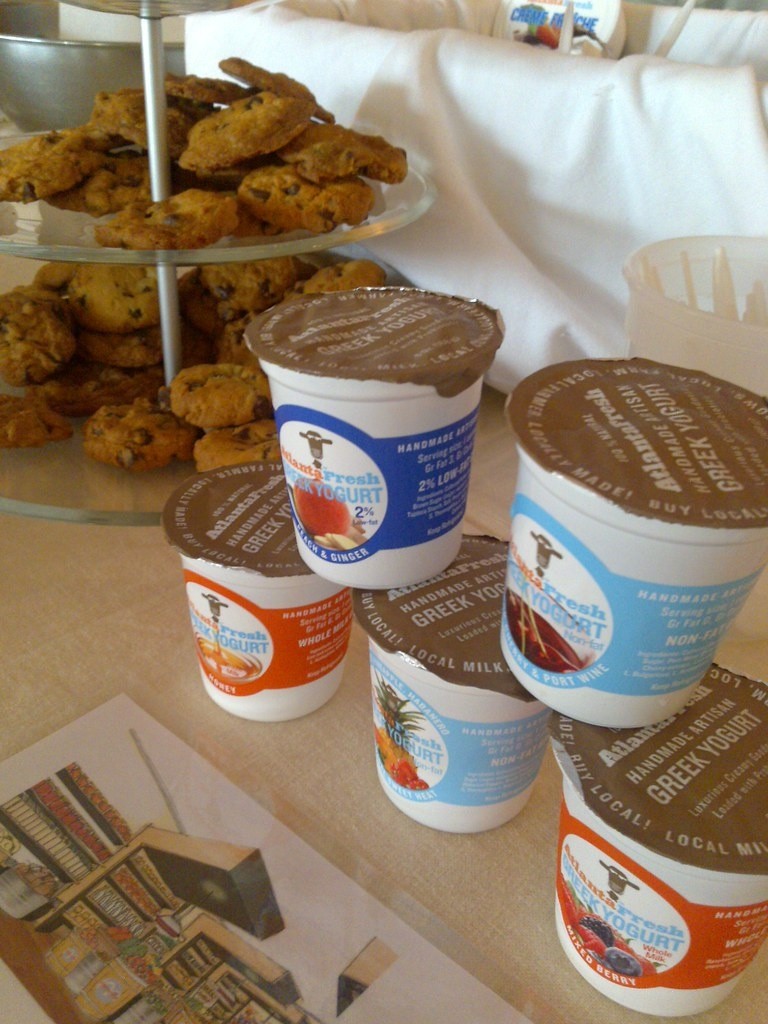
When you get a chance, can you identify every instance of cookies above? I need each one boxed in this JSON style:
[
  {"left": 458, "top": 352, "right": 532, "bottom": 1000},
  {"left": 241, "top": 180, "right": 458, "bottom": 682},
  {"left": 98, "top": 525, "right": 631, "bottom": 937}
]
[
  {"left": 0, "top": 258, "right": 391, "bottom": 476},
  {"left": 0, "top": 54, "right": 407, "bottom": 250}
]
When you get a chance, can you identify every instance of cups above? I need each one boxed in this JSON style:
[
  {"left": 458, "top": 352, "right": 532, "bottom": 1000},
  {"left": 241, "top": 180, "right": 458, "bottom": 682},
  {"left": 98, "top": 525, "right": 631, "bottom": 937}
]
[{"left": 623, "top": 237, "right": 768, "bottom": 644}]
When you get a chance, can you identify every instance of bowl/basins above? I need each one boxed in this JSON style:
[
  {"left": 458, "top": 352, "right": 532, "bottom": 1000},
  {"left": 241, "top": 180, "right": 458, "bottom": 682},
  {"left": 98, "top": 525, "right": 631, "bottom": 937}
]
[{"left": 0, "top": 34, "right": 186, "bottom": 133}]
